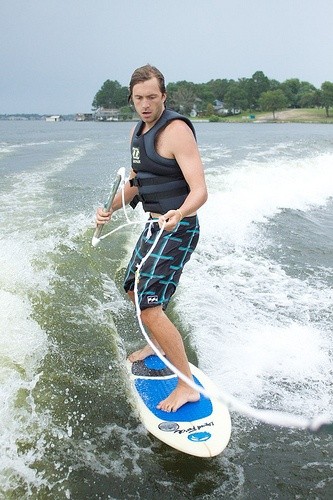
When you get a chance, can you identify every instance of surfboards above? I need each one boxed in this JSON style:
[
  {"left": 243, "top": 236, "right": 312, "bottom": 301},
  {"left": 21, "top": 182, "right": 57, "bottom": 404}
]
[{"left": 106, "top": 286, "right": 233, "bottom": 459}]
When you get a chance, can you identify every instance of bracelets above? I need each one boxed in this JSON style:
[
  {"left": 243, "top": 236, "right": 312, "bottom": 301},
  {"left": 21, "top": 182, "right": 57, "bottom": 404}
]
[{"left": 175, "top": 207, "right": 186, "bottom": 222}]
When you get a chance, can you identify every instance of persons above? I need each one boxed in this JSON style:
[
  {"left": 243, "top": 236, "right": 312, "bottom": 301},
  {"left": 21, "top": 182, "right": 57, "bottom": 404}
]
[{"left": 96, "top": 64, "right": 209, "bottom": 413}]
